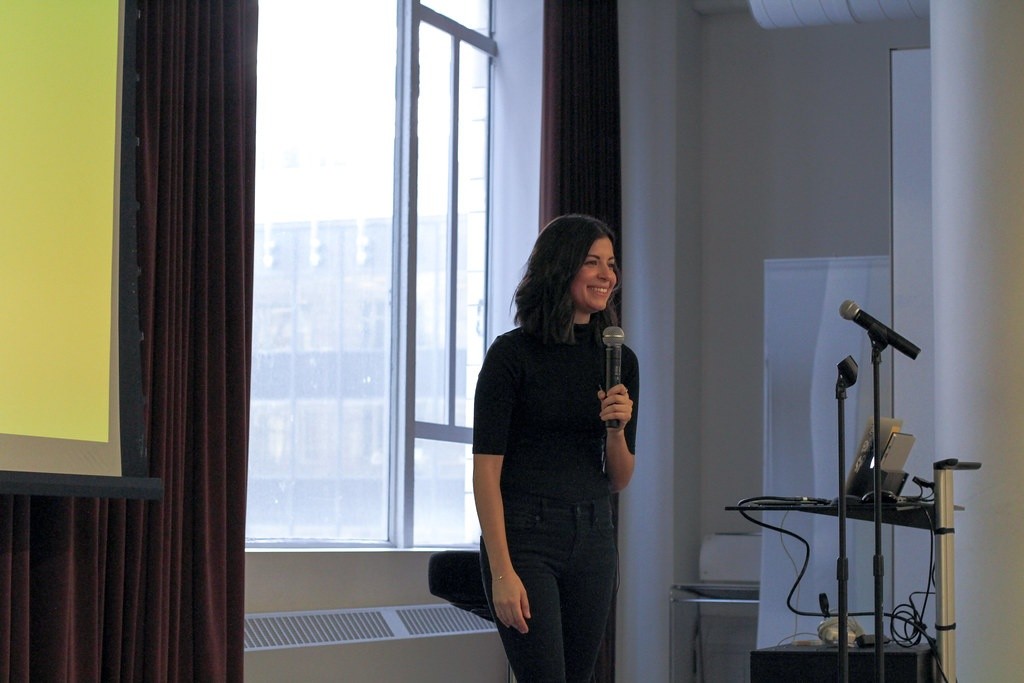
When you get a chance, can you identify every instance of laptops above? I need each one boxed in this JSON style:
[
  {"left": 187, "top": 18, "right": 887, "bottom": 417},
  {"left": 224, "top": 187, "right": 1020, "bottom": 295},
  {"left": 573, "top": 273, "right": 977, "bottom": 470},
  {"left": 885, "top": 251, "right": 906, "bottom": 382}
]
[{"left": 752, "top": 417, "right": 904, "bottom": 506}]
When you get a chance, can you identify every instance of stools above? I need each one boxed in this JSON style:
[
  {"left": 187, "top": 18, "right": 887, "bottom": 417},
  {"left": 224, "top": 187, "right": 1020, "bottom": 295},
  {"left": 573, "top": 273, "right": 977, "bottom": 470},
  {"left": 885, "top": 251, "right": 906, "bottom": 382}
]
[{"left": 428, "top": 550, "right": 517, "bottom": 683}]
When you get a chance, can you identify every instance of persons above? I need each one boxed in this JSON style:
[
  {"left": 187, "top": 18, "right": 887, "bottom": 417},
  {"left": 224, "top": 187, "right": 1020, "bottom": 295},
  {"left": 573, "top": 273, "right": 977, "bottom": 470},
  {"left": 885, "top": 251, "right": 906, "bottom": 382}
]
[{"left": 472, "top": 213, "right": 640, "bottom": 683}]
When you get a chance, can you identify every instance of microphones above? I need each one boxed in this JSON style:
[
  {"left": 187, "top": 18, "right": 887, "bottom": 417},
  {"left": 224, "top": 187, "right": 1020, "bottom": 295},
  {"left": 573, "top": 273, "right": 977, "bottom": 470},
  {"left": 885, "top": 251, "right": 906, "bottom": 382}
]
[
  {"left": 839, "top": 300, "right": 922, "bottom": 360},
  {"left": 602, "top": 326, "right": 625, "bottom": 428}
]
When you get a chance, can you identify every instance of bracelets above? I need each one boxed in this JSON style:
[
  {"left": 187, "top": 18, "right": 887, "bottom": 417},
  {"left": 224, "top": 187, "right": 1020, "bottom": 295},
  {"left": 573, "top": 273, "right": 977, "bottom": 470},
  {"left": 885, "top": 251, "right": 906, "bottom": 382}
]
[{"left": 492, "top": 569, "right": 514, "bottom": 580}]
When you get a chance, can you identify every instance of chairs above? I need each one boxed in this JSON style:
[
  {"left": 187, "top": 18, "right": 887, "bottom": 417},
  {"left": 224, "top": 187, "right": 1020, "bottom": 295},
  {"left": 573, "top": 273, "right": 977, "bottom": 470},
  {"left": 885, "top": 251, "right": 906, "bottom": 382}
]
[{"left": 667, "top": 532, "right": 763, "bottom": 683}]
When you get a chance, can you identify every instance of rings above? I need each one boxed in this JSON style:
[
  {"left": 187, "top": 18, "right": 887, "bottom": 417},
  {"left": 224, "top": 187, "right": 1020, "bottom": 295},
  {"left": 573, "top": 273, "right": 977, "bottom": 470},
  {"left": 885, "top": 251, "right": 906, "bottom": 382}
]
[{"left": 623, "top": 389, "right": 628, "bottom": 395}]
